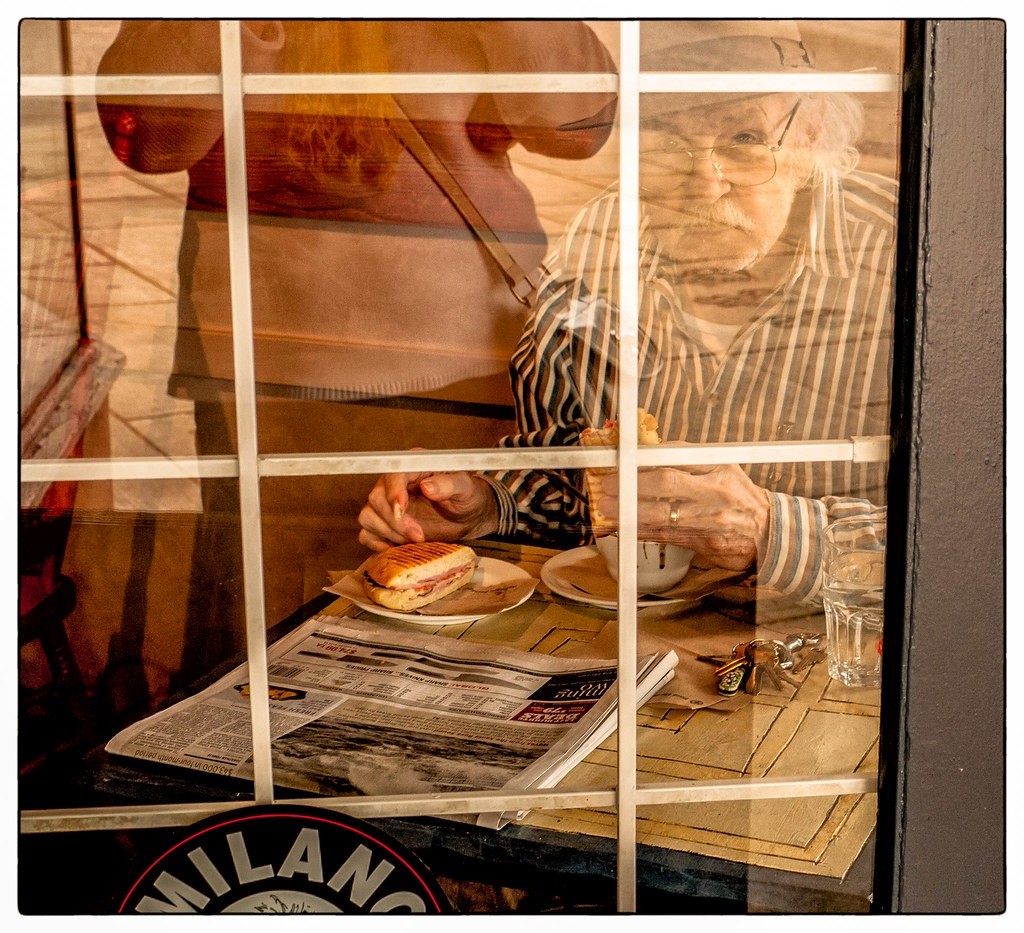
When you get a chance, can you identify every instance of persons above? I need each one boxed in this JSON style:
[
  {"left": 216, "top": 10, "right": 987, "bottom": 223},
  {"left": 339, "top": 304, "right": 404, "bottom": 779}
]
[
  {"left": 352, "top": 20, "right": 903, "bottom": 628},
  {"left": 98, "top": 21, "right": 618, "bottom": 694}
]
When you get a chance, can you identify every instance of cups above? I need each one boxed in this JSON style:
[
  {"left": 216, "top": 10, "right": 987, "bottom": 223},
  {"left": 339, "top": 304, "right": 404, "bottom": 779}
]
[
  {"left": 599, "top": 532, "right": 697, "bottom": 592},
  {"left": 818, "top": 517, "right": 886, "bottom": 688}
]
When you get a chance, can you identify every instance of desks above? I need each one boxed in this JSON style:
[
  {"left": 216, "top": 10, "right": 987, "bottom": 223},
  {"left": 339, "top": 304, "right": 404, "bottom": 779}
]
[{"left": 87, "top": 540, "right": 882, "bottom": 914}]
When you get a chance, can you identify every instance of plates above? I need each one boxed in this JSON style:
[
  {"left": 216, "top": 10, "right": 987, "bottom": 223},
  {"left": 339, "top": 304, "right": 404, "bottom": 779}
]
[
  {"left": 356, "top": 557, "right": 537, "bottom": 625},
  {"left": 541, "top": 545, "right": 684, "bottom": 611}
]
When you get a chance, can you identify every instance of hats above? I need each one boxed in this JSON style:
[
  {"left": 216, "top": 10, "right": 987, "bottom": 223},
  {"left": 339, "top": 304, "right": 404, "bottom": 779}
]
[{"left": 636, "top": 21, "right": 817, "bottom": 122}]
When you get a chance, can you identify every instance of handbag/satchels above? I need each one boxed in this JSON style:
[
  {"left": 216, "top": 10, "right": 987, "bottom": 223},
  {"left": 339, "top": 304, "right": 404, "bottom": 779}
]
[{"left": 524, "top": 279, "right": 660, "bottom": 430}]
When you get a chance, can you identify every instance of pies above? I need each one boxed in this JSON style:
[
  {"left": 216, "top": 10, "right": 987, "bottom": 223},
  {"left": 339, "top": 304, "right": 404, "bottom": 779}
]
[
  {"left": 579, "top": 408, "right": 663, "bottom": 537},
  {"left": 361, "top": 541, "right": 477, "bottom": 610},
  {"left": 273, "top": 20, "right": 398, "bottom": 199}
]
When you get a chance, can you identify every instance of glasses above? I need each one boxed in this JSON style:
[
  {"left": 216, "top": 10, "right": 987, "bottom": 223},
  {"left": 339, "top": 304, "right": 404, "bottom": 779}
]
[{"left": 636, "top": 97, "right": 806, "bottom": 195}]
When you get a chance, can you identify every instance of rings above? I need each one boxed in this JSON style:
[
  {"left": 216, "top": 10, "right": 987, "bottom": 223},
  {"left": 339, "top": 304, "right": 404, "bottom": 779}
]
[{"left": 669, "top": 502, "right": 682, "bottom": 526}]
